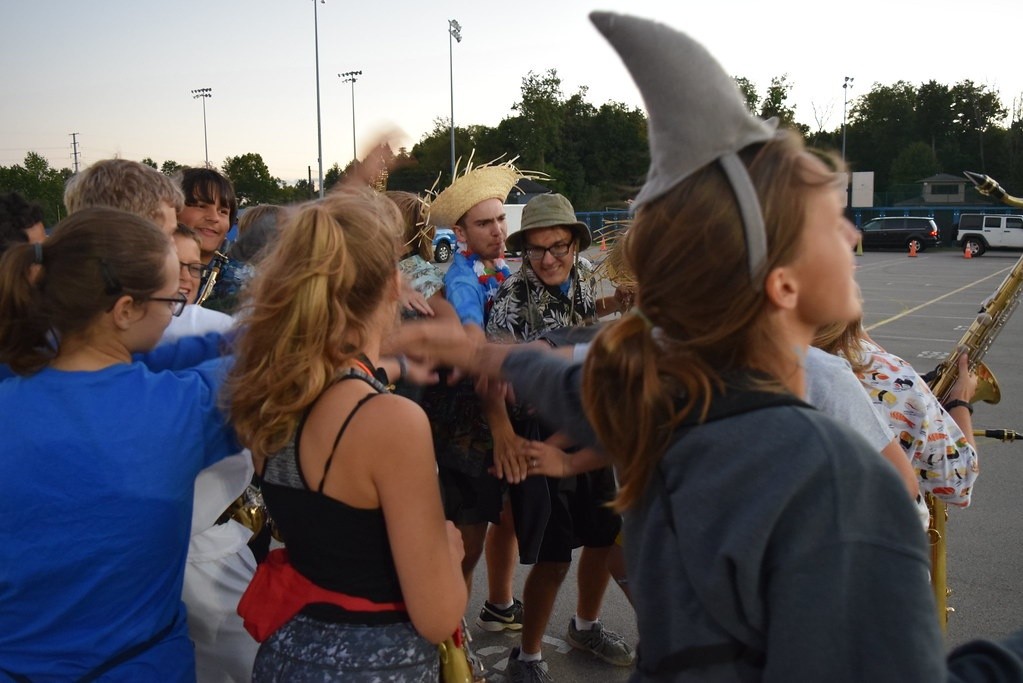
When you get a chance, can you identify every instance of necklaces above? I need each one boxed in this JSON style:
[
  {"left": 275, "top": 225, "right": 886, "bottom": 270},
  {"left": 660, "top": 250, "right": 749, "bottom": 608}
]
[{"left": 348, "top": 359, "right": 375, "bottom": 377}]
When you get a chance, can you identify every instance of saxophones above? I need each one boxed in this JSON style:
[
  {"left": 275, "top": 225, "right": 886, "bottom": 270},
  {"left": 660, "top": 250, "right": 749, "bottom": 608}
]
[
  {"left": 438, "top": 615, "right": 487, "bottom": 683},
  {"left": 929, "top": 170, "right": 1023, "bottom": 646},
  {"left": 225, "top": 484, "right": 287, "bottom": 543},
  {"left": 196, "top": 238, "right": 231, "bottom": 307}
]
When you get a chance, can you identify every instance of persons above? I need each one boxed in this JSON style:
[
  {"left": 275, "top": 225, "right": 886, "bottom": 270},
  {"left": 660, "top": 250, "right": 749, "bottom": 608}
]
[
  {"left": 0, "top": 156, "right": 636, "bottom": 683},
  {"left": 0, "top": 208, "right": 258, "bottom": 683},
  {"left": 426, "top": 8, "right": 980, "bottom": 683}
]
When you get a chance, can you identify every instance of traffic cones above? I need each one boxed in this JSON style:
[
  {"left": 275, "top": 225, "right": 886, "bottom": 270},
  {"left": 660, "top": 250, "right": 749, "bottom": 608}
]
[
  {"left": 908, "top": 240, "right": 917, "bottom": 257},
  {"left": 600, "top": 236, "right": 607, "bottom": 250},
  {"left": 963, "top": 240, "right": 972, "bottom": 259}
]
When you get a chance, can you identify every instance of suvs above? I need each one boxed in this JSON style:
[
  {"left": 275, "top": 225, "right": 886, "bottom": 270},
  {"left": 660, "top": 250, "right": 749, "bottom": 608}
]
[
  {"left": 854, "top": 217, "right": 943, "bottom": 252},
  {"left": 957, "top": 213, "right": 1023, "bottom": 257}
]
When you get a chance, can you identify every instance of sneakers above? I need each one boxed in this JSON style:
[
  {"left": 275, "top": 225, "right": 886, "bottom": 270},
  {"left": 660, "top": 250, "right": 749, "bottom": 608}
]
[
  {"left": 566, "top": 616, "right": 636, "bottom": 665},
  {"left": 504, "top": 646, "right": 555, "bottom": 683},
  {"left": 475, "top": 597, "right": 525, "bottom": 632}
]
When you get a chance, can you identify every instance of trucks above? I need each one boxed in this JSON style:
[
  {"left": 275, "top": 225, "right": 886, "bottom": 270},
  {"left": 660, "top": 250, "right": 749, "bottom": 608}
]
[{"left": 431, "top": 204, "right": 527, "bottom": 263}]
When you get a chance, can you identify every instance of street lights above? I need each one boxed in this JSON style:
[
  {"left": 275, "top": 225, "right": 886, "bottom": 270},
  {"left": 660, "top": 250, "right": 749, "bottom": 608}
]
[
  {"left": 312, "top": 0, "right": 326, "bottom": 199},
  {"left": 338, "top": 71, "right": 362, "bottom": 167},
  {"left": 191, "top": 88, "right": 212, "bottom": 168},
  {"left": 449, "top": 19, "right": 462, "bottom": 175}
]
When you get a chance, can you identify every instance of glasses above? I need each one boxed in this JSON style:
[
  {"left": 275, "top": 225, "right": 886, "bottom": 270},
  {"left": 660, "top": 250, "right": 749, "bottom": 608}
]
[
  {"left": 179, "top": 261, "right": 209, "bottom": 278},
  {"left": 106, "top": 291, "right": 188, "bottom": 317},
  {"left": 523, "top": 234, "right": 577, "bottom": 259}
]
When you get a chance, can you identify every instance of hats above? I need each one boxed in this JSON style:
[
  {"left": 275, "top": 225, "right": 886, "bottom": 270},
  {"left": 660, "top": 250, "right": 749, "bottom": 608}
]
[
  {"left": 404, "top": 149, "right": 557, "bottom": 246},
  {"left": 505, "top": 194, "right": 592, "bottom": 253}
]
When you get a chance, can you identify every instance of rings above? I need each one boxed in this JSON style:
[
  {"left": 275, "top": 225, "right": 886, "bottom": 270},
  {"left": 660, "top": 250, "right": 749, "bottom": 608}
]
[{"left": 533, "top": 459, "right": 536, "bottom": 466}]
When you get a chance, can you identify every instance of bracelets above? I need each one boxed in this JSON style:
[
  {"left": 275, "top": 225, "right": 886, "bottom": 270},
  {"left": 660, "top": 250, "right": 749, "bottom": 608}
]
[
  {"left": 944, "top": 399, "right": 974, "bottom": 416},
  {"left": 397, "top": 353, "right": 408, "bottom": 384}
]
